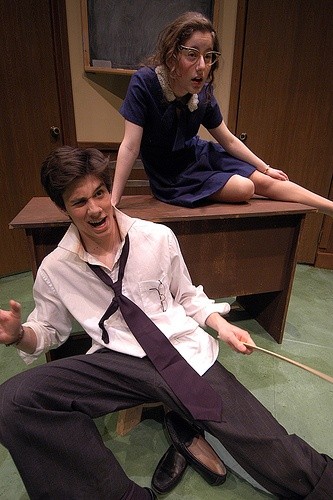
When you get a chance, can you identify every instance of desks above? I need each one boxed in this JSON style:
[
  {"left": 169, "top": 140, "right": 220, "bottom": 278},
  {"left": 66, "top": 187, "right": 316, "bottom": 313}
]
[{"left": 11, "top": 194, "right": 317, "bottom": 363}]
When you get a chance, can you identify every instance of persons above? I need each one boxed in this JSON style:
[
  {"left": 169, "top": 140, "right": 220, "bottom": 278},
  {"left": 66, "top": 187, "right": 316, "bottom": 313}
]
[
  {"left": 1, "top": 143, "right": 333, "bottom": 500},
  {"left": 110, "top": 13, "right": 333, "bottom": 217}
]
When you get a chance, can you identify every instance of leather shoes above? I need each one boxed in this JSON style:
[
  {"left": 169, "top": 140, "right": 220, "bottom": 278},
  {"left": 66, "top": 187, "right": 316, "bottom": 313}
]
[
  {"left": 162, "top": 409, "right": 227, "bottom": 486},
  {"left": 151, "top": 446, "right": 188, "bottom": 495}
]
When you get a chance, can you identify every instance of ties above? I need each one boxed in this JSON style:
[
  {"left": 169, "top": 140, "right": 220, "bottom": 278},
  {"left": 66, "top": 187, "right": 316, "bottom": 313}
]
[{"left": 86, "top": 233, "right": 221, "bottom": 422}]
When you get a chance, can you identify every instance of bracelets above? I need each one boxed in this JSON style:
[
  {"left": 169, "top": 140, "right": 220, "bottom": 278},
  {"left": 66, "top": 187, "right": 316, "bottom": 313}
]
[
  {"left": 4, "top": 324, "right": 25, "bottom": 347},
  {"left": 262, "top": 165, "right": 270, "bottom": 174}
]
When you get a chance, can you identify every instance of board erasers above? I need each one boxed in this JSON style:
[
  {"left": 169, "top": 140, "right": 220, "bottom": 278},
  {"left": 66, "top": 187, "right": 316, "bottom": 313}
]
[{"left": 92, "top": 60, "right": 111, "bottom": 68}]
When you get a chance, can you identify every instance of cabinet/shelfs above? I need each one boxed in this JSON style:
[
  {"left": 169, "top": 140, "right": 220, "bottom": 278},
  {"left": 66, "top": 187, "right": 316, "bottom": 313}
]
[
  {"left": 227, "top": 0, "right": 333, "bottom": 269},
  {"left": 0, "top": 0, "right": 75, "bottom": 278}
]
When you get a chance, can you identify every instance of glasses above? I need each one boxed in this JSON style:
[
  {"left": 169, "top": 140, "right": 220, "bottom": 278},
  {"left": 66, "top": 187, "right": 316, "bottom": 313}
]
[{"left": 177, "top": 44, "right": 222, "bottom": 66}]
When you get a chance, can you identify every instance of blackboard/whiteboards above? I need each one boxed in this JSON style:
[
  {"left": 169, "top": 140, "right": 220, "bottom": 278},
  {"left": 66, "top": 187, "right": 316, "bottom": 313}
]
[{"left": 80, "top": 0, "right": 220, "bottom": 76}]
